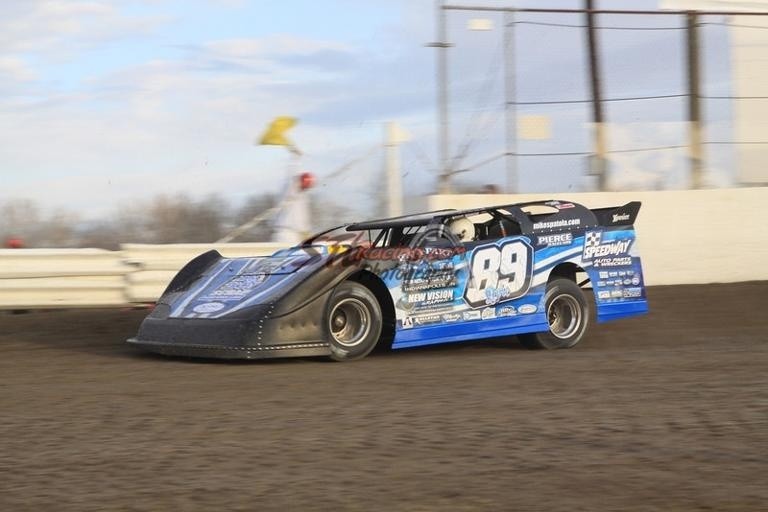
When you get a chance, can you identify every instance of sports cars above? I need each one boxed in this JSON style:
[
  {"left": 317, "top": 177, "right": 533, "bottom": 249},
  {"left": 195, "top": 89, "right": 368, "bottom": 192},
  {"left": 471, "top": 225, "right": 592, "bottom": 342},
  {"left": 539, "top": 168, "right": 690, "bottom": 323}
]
[{"left": 122, "top": 196, "right": 649, "bottom": 365}]
